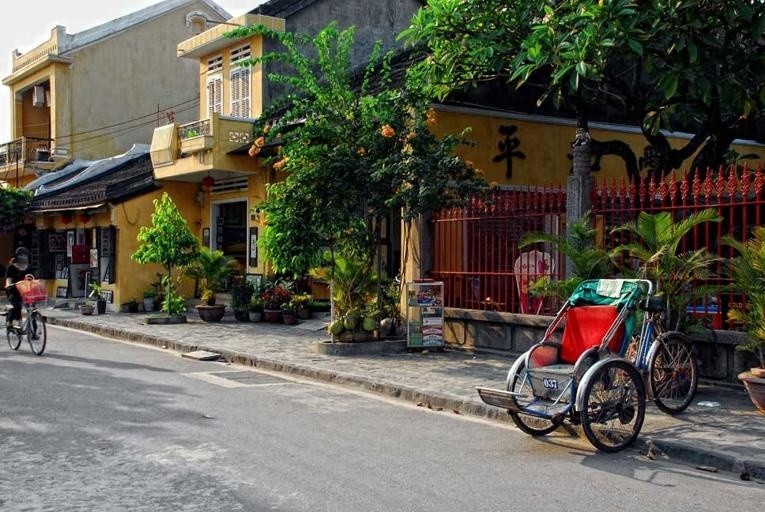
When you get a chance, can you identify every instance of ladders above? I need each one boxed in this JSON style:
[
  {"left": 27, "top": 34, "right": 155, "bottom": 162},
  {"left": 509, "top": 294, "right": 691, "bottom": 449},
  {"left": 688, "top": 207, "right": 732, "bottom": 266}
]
[{"left": 31, "top": 229, "right": 40, "bottom": 279}]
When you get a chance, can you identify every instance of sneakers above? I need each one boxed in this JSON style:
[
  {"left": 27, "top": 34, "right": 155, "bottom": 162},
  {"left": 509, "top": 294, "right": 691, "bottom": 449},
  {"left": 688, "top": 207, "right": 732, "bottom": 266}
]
[{"left": 12, "top": 319, "right": 21, "bottom": 328}]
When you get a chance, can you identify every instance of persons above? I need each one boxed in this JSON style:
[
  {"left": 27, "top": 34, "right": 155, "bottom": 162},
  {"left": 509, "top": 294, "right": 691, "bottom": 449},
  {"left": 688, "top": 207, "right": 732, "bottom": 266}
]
[
  {"left": 49, "top": 228, "right": 109, "bottom": 291},
  {"left": 3, "top": 246, "right": 41, "bottom": 341}
]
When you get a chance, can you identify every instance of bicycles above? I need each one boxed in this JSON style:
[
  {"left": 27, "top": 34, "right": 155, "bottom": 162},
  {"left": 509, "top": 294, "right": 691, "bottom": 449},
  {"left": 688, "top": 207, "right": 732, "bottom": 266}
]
[{"left": 4, "top": 283, "right": 46, "bottom": 356}]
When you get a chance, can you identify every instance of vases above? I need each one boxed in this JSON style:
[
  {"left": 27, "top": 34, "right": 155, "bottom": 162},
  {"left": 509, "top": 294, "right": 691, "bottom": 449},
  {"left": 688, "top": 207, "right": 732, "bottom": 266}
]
[
  {"left": 235, "top": 309, "right": 311, "bottom": 325},
  {"left": 128, "top": 303, "right": 139, "bottom": 313},
  {"left": 97, "top": 300, "right": 106, "bottom": 314},
  {"left": 232, "top": 281, "right": 313, "bottom": 314},
  {"left": 79, "top": 306, "right": 96, "bottom": 315}
]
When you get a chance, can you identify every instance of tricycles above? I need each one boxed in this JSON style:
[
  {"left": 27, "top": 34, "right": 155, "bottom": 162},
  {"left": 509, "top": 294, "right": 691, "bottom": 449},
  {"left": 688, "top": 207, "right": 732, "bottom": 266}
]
[{"left": 475, "top": 277, "right": 700, "bottom": 454}]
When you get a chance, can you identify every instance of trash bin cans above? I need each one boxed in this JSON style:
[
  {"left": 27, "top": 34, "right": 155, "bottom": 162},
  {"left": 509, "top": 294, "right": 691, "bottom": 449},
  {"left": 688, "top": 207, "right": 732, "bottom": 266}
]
[{"left": 686, "top": 292, "right": 722, "bottom": 329}]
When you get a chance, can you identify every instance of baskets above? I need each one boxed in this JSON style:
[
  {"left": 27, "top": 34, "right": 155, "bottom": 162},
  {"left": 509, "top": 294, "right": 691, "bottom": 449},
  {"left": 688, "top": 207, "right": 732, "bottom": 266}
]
[{"left": 14, "top": 274, "right": 48, "bottom": 304}]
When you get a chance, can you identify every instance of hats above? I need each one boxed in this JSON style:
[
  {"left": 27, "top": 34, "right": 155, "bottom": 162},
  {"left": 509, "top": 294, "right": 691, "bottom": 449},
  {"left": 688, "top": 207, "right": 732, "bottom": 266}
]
[{"left": 15, "top": 247, "right": 30, "bottom": 255}]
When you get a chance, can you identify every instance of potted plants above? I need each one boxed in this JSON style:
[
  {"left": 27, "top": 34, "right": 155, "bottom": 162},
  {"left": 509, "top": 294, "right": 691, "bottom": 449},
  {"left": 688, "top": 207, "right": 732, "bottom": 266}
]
[
  {"left": 719, "top": 226, "right": 765, "bottom": 413},
  {"left": 179, "top": 247, "right": 242, "bottom": 323},
  {"left": 144, "top": 291, "right": 157, "bottom": 312},
  {"left": 610, "top": 209, "right": 723, "bottom": 399}
]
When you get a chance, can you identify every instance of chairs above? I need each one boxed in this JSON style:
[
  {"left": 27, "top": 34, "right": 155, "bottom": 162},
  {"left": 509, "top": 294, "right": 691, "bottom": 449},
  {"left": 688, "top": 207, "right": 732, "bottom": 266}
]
[{"left": 525, "top": 305, "right": 624, "bottom": 404}]
